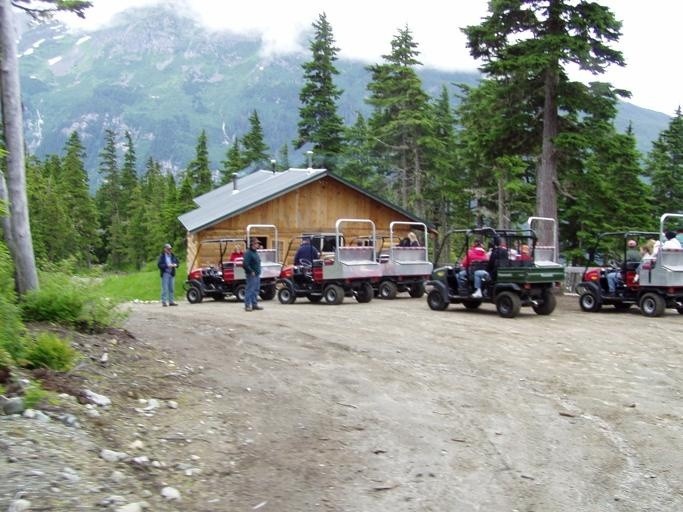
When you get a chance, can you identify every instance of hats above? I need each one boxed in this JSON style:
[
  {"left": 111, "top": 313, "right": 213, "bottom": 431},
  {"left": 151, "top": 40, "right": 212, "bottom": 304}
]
[
  {"left": 250, "top": 237, "right": 262, "bottom": 245},
  {"left": 627, "top": 239, "right": 637, "bottom": 247},
  {"left": 165, "top": 244, "right": 172, "bottom": 249}
]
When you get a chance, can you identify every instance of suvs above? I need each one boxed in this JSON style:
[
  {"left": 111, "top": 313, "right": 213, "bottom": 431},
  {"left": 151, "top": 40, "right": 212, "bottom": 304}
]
[
  {"left": 422, "top": 218, "right": 564, "bottom": 318},
  {"left": 348, "top": 221, "right": 432, "bottom": 300},
  {"left": 183, "top": 224, "right": 282, "bottom": 304},
  {"left": 276, "top": 219, "right": 383, "bottom": 304},
  {"left": 575, "top": 213, "right": 683, "bottom": 316}
]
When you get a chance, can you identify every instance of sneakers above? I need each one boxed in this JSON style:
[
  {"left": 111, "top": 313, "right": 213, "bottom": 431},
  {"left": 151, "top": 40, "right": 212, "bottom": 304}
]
[
  {"left": 471, "top": 290, "right": 484, "bottom": 299},
  {"left": 163, "top": 302, "right": 179, "bottom": 306},
  {"left": 245, "top": 304, "right": 264, "bottom": 311}
]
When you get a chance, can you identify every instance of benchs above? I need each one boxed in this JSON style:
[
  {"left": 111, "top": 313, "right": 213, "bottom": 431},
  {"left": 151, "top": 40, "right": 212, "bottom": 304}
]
[
  {"left": 325, "top": 240, "right": 372, "bottom": 265},
  {"left": 391, "top": 245, "right": 427, "bottom": 263},
  {"left": 617, "top": 261, "right": 639, "bottom": 287},
  {"left": 322, "top": 252, "right": 336, "bottom": 265},
  {"left": 489, "top": 258, "right": 534, "bottom": 284},
  {"left": 467, "top": 260, "right": 488, "bottom": 283},
  {"left": 233, "top": 249, "right": 282, "bottom": 280},
  {"left": 638, "top": 247, "right": 683, "bottom": 287},
  {"left": 378, "top": 253, "right": 389, "bottom": 264}
]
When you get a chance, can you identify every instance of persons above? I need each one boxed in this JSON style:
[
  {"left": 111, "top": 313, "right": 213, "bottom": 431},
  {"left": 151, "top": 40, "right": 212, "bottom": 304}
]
[
  {"left": 293, "top": 238, "right": 320, "bottom": 266},
  {"left": 605, "top": 228, "right": 682, "bottom": 297},
  {"left": 240, "top": 237, "right": 264, "bottom": 312},
  {"left": 156, "top": 243, "right": 180, "bottom": 308},
  {"left": 459, "top": 237, "right": 534, "bottom": 298},
  {"left": 408, "top": 232, "right": 419, "bottom": 246},
  {"left": 228, "top": 245, "right": 244, "bottom": 261}
]
